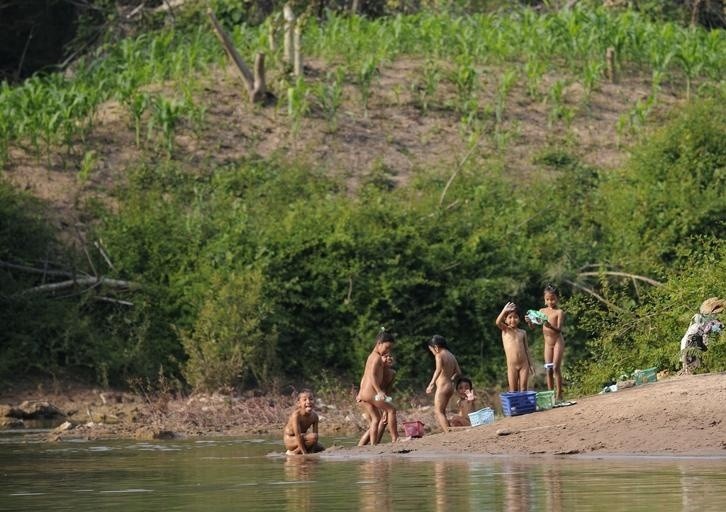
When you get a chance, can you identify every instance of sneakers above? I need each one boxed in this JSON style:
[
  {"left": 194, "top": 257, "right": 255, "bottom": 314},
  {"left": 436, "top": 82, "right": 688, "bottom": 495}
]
[{"left": 552, "top": 399, "right": 576, "bottom": 408}]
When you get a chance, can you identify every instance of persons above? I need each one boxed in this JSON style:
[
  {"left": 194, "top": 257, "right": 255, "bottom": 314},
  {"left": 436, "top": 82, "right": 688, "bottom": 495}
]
[
  {"left": 495, "top": 301, "right": 536, "bottom": 393},
  {"left": 283, "top": 388, "right": 326, "bottom": 456},
  {"left": 356, "top": 330, "right": 399, "bottom": 446},
  {"left": 423, "top": 335, "right": 477, "bottom": 435},
  {"left": 525, "top": 285, "right": 565, "bottom": 403}
]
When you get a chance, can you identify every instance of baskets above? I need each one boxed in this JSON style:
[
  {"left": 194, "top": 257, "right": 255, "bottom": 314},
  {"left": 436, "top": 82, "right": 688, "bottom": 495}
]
[
  {"left": 536, "top": 391, "right": 555, "bottom": 412},
  {"left": 631, "top": 367, "right": 657, "bottom": 385},
  {"left": 468, "top": 407, "right": 495, "bottom": 427},
  {"left": 499, "top": 390, "right": 537, "bottom": 416},
  {"left": 402, "top": 421, "right": 425, "bottom": 437}
]
